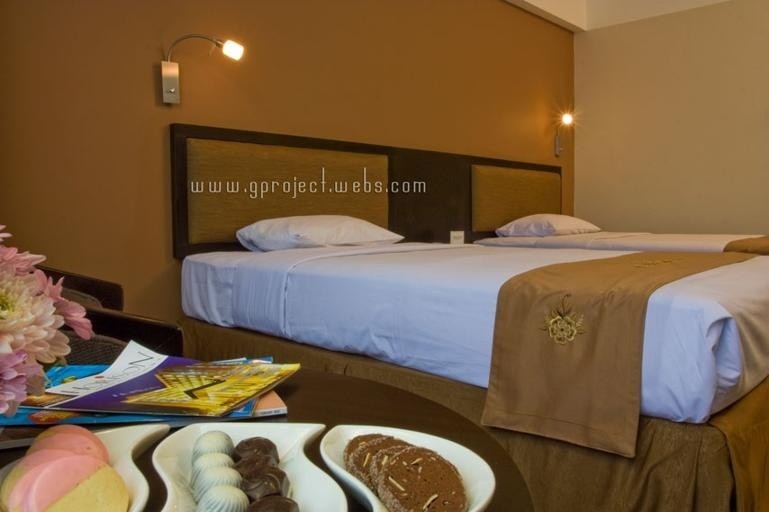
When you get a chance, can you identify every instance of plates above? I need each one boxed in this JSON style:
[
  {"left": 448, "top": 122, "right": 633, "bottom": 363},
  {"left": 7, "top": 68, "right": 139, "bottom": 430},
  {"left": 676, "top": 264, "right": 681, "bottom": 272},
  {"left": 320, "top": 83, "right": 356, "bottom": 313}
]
[
  {"left": 150, "top": 421, "right": 348, "bottom": 512},
  {"left": 1, "top": 424, "right": 170, "bottom": 512},
  {"left": 319, "top": 421, "right": 497, "bottom": 512}
]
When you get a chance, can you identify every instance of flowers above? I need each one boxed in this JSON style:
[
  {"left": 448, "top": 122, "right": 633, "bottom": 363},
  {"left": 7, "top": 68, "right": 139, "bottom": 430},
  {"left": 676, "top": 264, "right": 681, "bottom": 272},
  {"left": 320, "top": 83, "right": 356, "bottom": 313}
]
[{"left": 0, "top": 224, "right": 96, "bottom": 418}]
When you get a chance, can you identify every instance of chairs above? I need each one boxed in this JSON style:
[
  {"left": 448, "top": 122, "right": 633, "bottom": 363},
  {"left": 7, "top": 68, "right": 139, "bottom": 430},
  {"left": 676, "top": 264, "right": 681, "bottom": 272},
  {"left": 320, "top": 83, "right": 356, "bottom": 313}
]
[{"left": 29, "top": 264, "right": 184, "bottom": 372}]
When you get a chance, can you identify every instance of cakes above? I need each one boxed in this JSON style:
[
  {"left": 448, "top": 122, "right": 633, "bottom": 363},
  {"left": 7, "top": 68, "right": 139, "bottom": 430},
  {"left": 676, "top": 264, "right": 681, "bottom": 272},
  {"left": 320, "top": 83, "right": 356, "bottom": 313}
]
[
  {"left": 189, "top": 430, "right": 301, "bottom": 512},
  {"left": 0, "top": 424, "right": 129, "bottom": 512}
]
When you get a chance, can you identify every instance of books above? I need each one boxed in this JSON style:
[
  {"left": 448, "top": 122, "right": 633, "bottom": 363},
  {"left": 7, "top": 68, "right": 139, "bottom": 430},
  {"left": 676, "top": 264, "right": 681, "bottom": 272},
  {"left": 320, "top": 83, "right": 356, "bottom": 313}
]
[{"left": 0, "top": 338, "right": 303, "bottom": 449}]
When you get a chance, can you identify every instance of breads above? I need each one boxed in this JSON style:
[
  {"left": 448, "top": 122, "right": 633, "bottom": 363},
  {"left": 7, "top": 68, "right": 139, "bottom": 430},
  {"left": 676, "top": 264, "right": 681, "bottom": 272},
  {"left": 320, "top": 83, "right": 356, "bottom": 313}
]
[{"left": 343, "top": 434, "right": 469, "bottom": 512}]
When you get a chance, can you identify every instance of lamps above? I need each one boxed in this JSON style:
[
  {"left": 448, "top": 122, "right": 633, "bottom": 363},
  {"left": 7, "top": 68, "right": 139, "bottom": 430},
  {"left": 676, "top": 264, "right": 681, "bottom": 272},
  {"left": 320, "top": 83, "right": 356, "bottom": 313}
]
[
  {"left": 161, "top": 34, "right": 244, "bottom": 103},
  {"left": 555, "top": 114, "right": 572, "bottom": 157}
]
